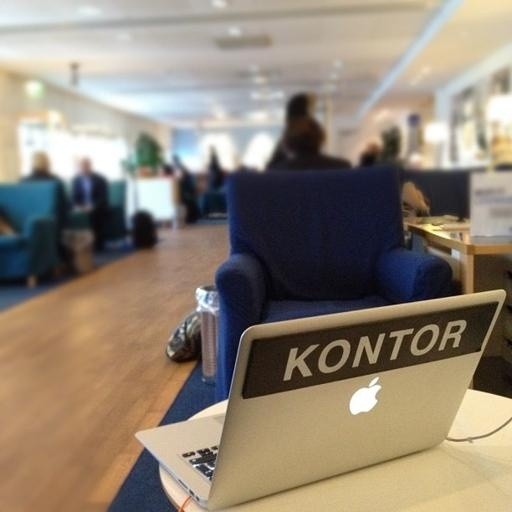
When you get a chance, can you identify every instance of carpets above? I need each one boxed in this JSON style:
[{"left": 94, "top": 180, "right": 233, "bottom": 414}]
[
  {"left": 107, "top": 355, "right": 213, "bottom": 512},
  {"left": 0, "top": 240, "right": 136, "bottom": 312}
]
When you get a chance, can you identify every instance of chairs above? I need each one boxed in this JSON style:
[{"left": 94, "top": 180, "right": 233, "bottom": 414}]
[
  {"left": 0, "top": 179, "right": 125, "bottom": 287},
  {"left": 214, "top": 162, "right": 454, "bottom": 404}
]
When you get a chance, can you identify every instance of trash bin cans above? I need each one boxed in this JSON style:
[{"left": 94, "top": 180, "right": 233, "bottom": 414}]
[{"left": 63, "top": 228, "right": 93, "bottom": 274}]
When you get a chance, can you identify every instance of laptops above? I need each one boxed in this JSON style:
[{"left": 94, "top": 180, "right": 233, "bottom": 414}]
[{"left": 134, "top": 289, "right": 507, "bottom": 510}]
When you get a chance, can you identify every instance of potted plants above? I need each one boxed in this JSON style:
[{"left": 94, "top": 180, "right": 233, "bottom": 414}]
[{"left": 135, "top": 132, "right": 164, "bottom": 177}]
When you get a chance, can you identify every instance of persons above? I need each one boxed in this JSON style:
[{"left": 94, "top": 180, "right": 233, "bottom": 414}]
[
  {"left": 0, "top": 150, "right": 112, "bottom": 288},
  {"left": 265, "top": 92, "right": 404, "bottom": 173},
  {"left": 138, "top": 144, "right": 222, "bottom": 224}
]
